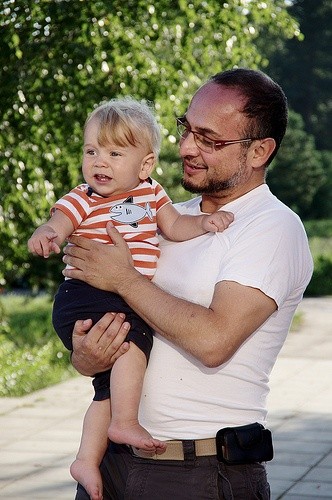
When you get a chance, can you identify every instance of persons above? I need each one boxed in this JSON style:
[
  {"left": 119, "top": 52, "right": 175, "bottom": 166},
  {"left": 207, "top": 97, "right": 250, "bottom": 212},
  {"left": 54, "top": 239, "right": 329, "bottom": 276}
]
[
  {"left": 60, "top": 67, "right": 315, "bottom": 499},
  {"left": 25, "top": 101, "right": 234, "bottom": 499}
]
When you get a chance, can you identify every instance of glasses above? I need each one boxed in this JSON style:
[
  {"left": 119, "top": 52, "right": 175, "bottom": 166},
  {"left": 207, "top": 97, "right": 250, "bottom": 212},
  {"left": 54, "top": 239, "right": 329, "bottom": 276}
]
[{"left": 176, "top": 117, "right": 260, "bottom": 153}]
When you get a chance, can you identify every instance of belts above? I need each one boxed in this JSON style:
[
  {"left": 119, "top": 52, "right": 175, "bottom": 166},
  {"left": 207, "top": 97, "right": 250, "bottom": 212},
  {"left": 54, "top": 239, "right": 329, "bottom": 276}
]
[{"left": 126, "top": 437, "right": 217, "bottom": 460}]
